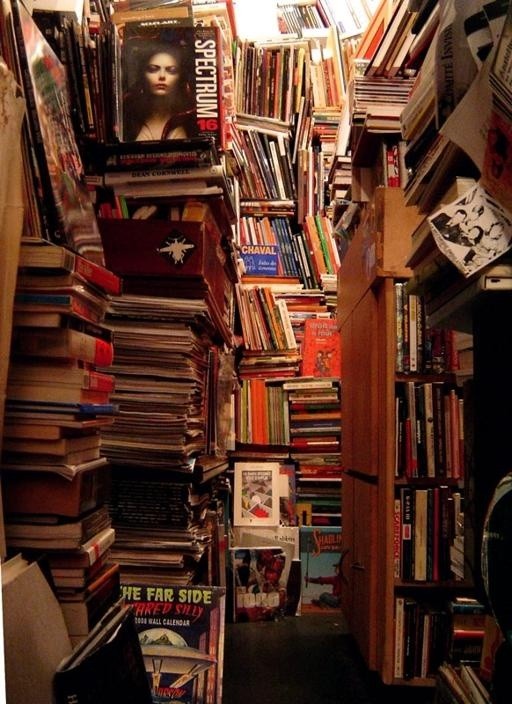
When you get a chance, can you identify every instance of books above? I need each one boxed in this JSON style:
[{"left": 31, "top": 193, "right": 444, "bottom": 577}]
[{"left": 0, "top": 0, "right": 512, "bottom": 704}]
